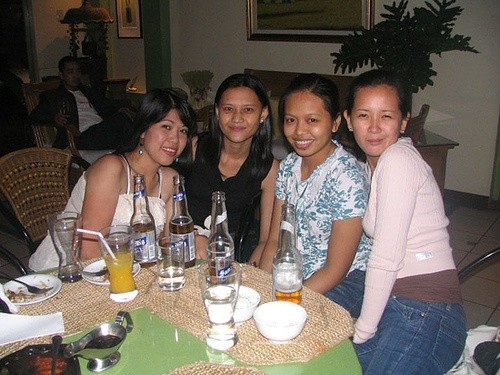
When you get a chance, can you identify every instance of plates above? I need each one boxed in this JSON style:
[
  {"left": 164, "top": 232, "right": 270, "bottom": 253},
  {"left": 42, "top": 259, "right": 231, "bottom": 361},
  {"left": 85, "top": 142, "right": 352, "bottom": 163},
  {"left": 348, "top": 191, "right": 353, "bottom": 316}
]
[
  {"left": 4, "top": 274, "right": 63, "bottom": 305},
  {"left": 81, "top": 257, "right": 141, "bottom": 286},
  {"left": 0, "top": 342, "right": 82, "bottom": 375}
]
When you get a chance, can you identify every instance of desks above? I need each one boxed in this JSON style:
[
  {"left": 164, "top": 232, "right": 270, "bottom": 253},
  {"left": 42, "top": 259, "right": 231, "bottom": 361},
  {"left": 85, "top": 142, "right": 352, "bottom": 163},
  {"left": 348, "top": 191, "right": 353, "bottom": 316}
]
[{"left": 407, "top": 129, "right": 459, "bottom": 201}]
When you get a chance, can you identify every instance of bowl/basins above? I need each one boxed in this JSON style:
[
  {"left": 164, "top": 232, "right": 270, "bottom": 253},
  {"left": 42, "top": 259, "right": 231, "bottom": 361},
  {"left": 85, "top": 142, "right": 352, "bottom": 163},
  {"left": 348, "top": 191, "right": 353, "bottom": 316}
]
[
  {"left": 253, "top": 300, "right": 308, "bottom": 341},
  {"left": 225, "top": 284, "right": 261, "bottom": 323}
]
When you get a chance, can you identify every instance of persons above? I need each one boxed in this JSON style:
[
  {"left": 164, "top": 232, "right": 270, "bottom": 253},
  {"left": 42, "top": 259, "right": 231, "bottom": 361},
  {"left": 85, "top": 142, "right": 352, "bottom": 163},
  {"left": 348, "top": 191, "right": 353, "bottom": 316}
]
[
  {"left": 27, "top": 56, "right": 139, "bottom": 150},
  {"left": 259, "top": 73, "right": 372, "bottom": 322},
  {"left": 27, "top": 86, "right": 198, "bottom": 273},
  {"left": 170, "top": 71, "right": 280, "bottom": 269},
  {"left": 322, "top": 69, "right": 468, "bottom": 374}
]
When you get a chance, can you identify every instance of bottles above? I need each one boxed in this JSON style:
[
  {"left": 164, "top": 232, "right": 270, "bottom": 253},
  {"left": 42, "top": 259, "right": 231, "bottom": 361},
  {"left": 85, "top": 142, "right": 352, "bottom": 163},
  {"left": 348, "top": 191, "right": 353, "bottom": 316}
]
[
  {"left": 128, "top": 174, "right": 159, "bottom": 268},
  {"left": 207, "top": 191, "right": 235, "bottom": 285},
  {"left": 271, "top": 203, "right": 303, "bottom": 304},
  {"left": 168, "top": 175, "right": 197, "bottom": 269}
]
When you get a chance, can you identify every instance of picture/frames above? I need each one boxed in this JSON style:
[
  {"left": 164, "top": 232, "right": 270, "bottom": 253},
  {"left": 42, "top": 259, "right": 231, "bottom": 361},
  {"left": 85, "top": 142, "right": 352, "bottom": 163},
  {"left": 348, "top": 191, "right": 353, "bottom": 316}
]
[
  {"left": 115, "top": 0, "right": 143, "bottom": 39},
  {"left": 246, "top": 0, "right": 376, "bottom": 43}
]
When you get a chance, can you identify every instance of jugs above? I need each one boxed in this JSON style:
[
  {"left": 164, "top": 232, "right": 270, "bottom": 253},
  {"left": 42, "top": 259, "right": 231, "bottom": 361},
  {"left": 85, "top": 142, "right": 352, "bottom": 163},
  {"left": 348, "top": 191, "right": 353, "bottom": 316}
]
[{"left": 63, "top": 310, "right": 134, "bottom": 373}]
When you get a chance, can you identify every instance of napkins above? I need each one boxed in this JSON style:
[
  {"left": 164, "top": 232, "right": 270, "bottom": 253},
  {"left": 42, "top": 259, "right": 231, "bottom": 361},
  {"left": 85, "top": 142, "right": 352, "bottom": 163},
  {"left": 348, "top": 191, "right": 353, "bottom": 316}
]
[{"left": 0, "top": 313, "right": 65, "bottom": 346}]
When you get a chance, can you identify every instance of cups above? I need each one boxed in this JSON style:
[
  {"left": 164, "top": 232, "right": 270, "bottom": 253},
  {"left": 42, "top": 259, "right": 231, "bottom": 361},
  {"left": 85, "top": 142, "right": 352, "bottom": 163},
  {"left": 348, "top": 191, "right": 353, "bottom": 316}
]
[
  {"left": 47, "top": 211, "right": 85, "bottom": 283},
  {"left": 99, "top": 224, "right": 139, "bottom": 302},
  {"left": 154, "top": 236, "right": 187, "bottom": 292},
  {"left": 196, "top": 257, "right": 243, "bottom": 349}
]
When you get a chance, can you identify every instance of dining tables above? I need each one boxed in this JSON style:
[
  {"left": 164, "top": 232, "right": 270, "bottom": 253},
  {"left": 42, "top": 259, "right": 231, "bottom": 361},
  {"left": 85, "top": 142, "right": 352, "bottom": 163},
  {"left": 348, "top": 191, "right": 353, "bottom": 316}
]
[{"left": 0, "top": 254, "right": 361, "bottom": 375}]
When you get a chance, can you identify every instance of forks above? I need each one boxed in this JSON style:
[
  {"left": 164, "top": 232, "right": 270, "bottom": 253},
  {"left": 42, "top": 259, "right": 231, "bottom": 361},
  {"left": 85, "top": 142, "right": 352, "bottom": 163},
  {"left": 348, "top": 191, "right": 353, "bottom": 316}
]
[{"left": 0, "top": 270, "right": 54, "bottom": 294}]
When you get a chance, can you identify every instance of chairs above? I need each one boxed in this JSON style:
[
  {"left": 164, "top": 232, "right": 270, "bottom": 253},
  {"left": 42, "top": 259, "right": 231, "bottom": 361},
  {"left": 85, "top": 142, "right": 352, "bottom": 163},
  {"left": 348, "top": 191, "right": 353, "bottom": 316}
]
[
  {"left": 0, "top": 147, "right": 93, "bottom": 255},
  {"left": 21, "top": 77, "right": 137, "bottom": 170},
  {"left": 434, "top": 245, "right": 500, "bottom": 375}
]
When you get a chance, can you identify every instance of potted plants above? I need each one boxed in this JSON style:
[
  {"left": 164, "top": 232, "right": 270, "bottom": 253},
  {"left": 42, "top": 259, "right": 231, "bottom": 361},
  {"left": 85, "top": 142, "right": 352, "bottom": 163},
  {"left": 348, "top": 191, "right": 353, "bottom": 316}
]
[{"left": 180, "top": 70, "right": 214, "bottom": 110}]
